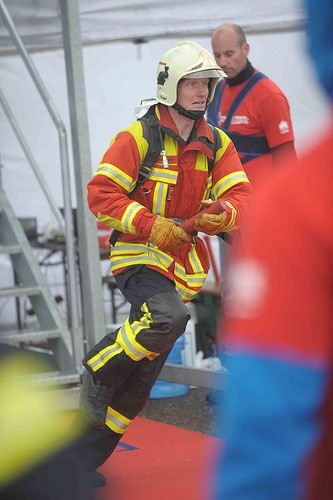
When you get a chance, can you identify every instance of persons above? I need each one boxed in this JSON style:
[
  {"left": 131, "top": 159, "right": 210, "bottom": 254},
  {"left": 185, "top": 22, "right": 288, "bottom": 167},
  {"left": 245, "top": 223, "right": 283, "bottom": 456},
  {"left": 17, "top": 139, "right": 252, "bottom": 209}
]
[
  {"left": 205, "top": 0, "right": 333, "bottom": 499},
  {"left": 71, "top": 40, "right": 255, "bottom": 486},
  {"left": 196, "top": 21, "right": 301, "bottom": 383},
  {"left": 0, "top": 337, "right": 112, "bottom": 500}
]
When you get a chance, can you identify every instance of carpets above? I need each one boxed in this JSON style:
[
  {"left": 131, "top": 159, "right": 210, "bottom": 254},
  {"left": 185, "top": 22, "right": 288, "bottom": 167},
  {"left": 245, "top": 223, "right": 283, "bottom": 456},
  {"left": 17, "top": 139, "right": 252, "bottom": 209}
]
[{"left": 93, "top": 415, "right": 222, "bottom": 500}]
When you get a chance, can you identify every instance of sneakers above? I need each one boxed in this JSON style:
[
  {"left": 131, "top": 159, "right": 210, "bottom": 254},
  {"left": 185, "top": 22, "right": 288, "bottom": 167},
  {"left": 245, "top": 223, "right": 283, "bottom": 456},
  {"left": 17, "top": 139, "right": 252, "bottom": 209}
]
[
  {"left": 90, "top": 471, "right": 105, "bottom": 488},
  {"left": 79, "top": 369, "right": 114, "bottom": 432}
]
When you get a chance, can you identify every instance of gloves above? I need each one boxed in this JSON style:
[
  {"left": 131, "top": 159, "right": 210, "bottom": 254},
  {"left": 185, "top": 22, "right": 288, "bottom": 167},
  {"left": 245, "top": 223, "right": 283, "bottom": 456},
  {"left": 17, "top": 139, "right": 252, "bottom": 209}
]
[
  {"left": 193, "top": 200, "right": 243, "bottom": 236},
  {"left": 136, "top": 212, "right": 191, "bottom": 253}
]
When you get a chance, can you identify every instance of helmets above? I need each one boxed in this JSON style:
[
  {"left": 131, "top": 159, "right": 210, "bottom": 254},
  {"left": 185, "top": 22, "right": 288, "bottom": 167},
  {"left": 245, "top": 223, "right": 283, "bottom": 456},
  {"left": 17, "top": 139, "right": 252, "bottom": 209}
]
[{"left": 156, "top": 41, "right": 228, "bottom": 107}]
